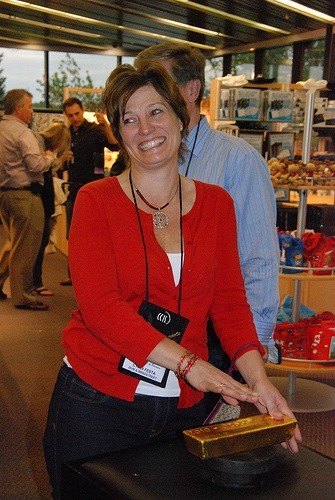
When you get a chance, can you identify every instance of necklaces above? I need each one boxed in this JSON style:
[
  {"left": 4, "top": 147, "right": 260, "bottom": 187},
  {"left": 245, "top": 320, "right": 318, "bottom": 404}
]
[
  {"left": 186, "top": 116, "right": 202, "bottom": 177},
  {"left": 132, "top": 183, "right": 179, "bottom": 229}
]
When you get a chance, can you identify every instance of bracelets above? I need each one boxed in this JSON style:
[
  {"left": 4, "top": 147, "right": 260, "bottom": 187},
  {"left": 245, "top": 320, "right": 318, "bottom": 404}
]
[{"left": 176, "top": 350, "right": 202, "bottom": 379}]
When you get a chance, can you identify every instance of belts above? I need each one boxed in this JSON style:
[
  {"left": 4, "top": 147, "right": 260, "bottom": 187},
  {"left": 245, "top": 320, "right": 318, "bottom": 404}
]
[{"left": 6, "top": 185, "right": 30, "bottom": 191}]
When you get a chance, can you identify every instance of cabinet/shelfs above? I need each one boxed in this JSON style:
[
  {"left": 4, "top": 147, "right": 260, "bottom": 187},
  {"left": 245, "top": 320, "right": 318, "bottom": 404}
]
[{"left": 209, "top": 81, "right": 335, "bottom": 414}]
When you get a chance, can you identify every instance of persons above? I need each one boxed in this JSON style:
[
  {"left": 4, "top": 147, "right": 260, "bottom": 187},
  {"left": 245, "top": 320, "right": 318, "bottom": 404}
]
[
  {"left": 29, "top": 97, "right": 126, "bottom": 296},
  {"left": 42, "top": 59, "right": 302, "bottom": 500},
  {"left": 0, "top": 89, "right": 54, "bottom": 310},
  {"left": 134, "top": 42, "right": 282, "bottom": 426}
]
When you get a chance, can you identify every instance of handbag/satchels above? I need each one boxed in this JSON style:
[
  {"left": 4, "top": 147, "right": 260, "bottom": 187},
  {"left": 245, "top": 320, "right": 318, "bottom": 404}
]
[
  {"left": 277, "top": 234, "right": 305, "bottom": 274},
  {"left": 298, "top": 232, "right": 335, "bottom": 274}
]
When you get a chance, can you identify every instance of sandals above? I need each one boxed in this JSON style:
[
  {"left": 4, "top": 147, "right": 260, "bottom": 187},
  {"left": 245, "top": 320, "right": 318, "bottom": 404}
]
[{"left": 33, "top": 285, "right": 54, "bottom": 296}]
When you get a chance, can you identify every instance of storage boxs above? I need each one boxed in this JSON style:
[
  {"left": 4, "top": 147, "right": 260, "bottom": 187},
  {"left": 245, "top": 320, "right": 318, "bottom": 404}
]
[
  {"left": 237, "top": 129, "right": 264, "bottom": 159},
  {"left": 220, "top": 88, "right": 294, "bottom": 121},
  {"left": 264, "top": 131, "right": 296, "bottom": 160}
]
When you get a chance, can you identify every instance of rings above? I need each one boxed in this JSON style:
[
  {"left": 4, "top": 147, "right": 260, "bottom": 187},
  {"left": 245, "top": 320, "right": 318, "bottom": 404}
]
[{"left": 219, "top": 382, "right": 225, "bottom": 388}]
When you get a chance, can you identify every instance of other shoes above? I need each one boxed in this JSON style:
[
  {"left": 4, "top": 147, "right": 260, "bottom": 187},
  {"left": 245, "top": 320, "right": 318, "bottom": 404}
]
[
  {"left": 59, "top": 278, "right": 72, "bottom": 286},
  {"left": 14, "top": 299, "right": 49, "bottom": 311}
]
